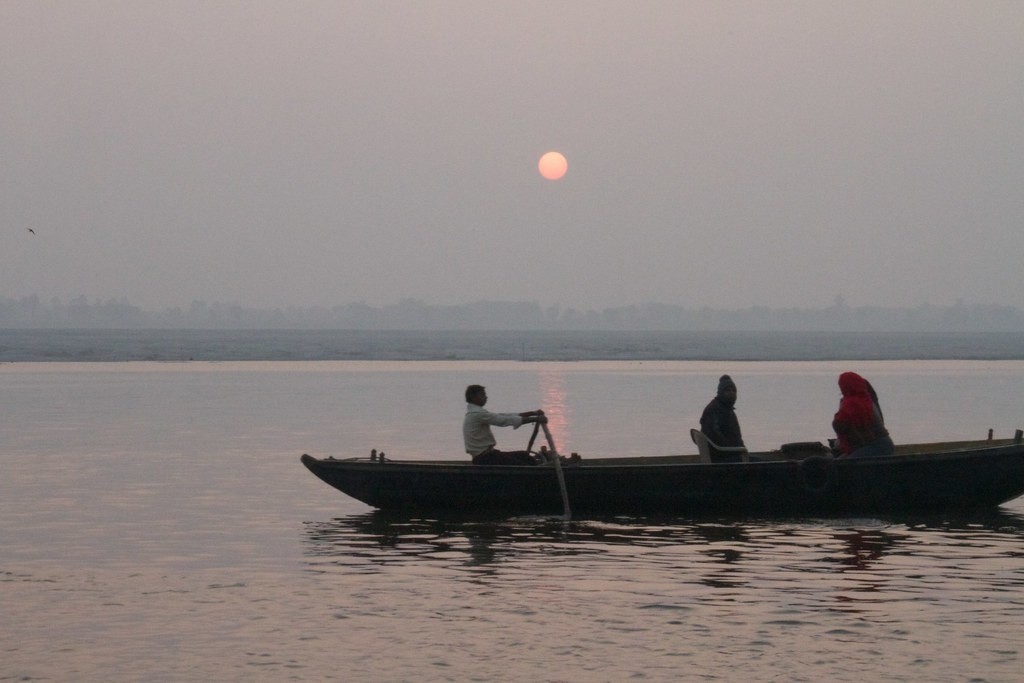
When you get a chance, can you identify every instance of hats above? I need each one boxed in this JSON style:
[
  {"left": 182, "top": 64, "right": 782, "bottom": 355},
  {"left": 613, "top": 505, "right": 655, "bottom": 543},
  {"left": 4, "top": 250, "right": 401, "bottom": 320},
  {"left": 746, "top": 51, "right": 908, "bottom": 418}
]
[{"left": 717, "top": 375, "right": 736, "bottom": 393}]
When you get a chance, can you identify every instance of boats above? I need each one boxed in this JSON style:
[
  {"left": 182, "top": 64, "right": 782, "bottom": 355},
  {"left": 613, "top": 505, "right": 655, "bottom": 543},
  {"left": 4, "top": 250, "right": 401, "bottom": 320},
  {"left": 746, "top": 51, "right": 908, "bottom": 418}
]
[{"left": 300, "top": 429, "right": 1024, "bottom": 515}]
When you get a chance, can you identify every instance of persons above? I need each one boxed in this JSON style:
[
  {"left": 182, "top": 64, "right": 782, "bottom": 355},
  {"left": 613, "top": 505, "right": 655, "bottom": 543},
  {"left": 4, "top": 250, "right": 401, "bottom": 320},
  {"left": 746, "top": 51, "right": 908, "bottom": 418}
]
[
  {"left": 463, "top": 385, "right": 548, "bottom": 466},
  {"left": 831, "top": 372, "right": 894, "bottom": 459},
  {"left": 699, "top": 374, "right": 780, "bottom": 461}
]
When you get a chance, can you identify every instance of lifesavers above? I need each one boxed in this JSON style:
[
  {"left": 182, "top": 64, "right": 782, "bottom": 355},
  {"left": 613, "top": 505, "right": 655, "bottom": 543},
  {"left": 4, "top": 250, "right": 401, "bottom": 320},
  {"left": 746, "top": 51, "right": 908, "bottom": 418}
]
[{"left": 796, "top": 456, "right": 840, "bottom": 494}]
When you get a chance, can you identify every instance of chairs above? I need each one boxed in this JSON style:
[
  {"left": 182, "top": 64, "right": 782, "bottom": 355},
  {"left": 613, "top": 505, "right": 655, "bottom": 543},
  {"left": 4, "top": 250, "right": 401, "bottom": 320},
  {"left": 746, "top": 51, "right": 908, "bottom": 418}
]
[{"left": 689, "top": 428, "right": 749, "bottom": 464}]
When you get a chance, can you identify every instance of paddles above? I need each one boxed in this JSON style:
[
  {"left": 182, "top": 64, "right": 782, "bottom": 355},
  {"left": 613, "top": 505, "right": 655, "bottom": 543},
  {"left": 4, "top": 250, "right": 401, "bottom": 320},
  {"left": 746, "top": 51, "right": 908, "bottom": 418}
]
[
  {"left": 526, "top": 414, "right": 541, "bottom": 457},
  {"left": 541, "top": 420, "right": 572, "bottom": 520}
]
